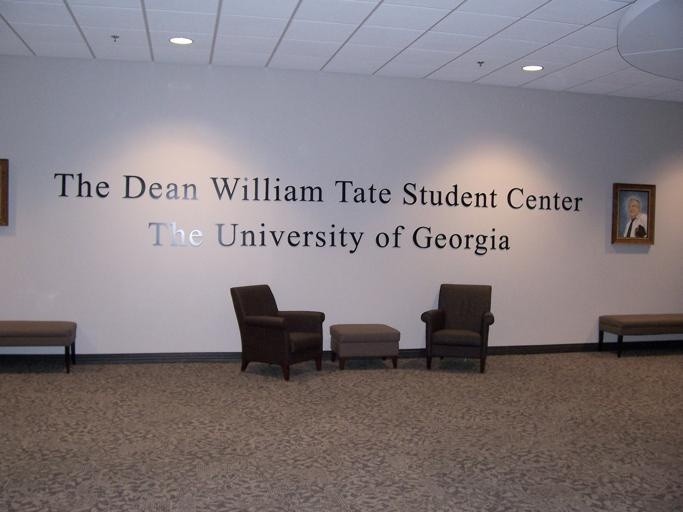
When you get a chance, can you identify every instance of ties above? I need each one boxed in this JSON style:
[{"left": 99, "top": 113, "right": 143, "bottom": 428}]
[{"left": 626, "top": 218, "right": 637, "bottom": 238}]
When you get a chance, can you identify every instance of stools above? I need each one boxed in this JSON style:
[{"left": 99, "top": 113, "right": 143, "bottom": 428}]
[{"left": 330, "top": 324, "right": 400, "bottom": 371}]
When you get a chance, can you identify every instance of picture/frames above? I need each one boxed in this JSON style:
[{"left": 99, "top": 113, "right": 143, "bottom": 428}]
[
  {"left": 1, "top": 158, "right": 9, "bottom": 226},
  {"left": 611, "top": 183, "right": 656, "bottom": 245}
]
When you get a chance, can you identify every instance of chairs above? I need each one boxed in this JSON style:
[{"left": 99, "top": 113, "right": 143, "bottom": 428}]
[
  {"left": 421, "top": 284, "right": 494, "bottom": 373},
  {"left": 230, "top": 285, "right": 324, "bottom": 381}
]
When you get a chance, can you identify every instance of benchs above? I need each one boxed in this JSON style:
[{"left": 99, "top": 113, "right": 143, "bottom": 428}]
[
  {"left": 0, "top": 320, "right": 77, "bottom": 373},
  {"left": 598, "top": 314, "right": 683, "bottom": 358}
]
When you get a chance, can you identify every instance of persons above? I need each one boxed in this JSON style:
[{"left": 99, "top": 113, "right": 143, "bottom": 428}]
[{"left": 622, "top": 197, "right": 647, "bottom": 238}]
